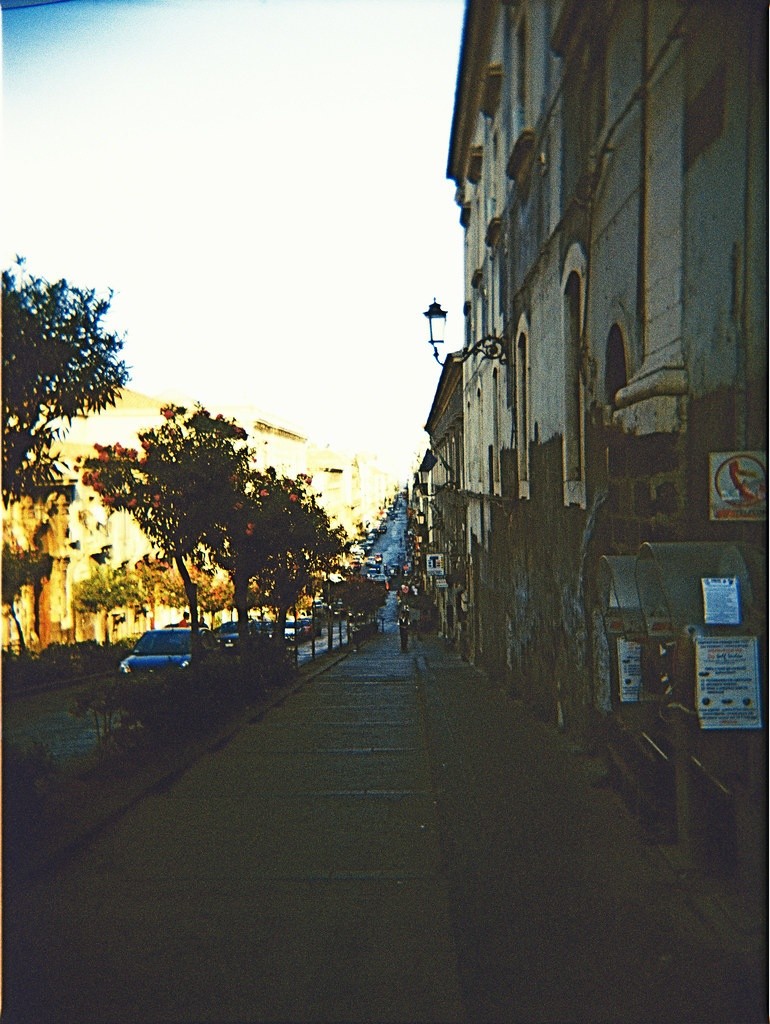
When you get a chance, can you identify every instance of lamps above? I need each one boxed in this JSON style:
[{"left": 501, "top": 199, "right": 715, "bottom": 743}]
[{"left": 424, "top": 296, "right": 506, "bottom": 367}]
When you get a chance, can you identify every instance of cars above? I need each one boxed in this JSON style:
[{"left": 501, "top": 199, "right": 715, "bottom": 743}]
[
  {"left": 309, "top": 598, "right": 365, "bottom": 622},
  {"left": 117, "top": 628, "right": 226, "bottom": 697},
  {"left": 346, "top": 525, "right": 387, "bottom": 585},
  {"left": 267, "top": 616, "right": 322, "bottom": 643},
  {"left": 215, "top": 620, "right": 262, "bottom": 651}
]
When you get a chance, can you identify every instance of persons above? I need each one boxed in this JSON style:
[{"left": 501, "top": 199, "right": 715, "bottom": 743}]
[
  {"left": 178, "top": 611, "right": 208, "bottom": 629},
  {"left": 396, "top": 611, "right": 410, "bottom": 654}
]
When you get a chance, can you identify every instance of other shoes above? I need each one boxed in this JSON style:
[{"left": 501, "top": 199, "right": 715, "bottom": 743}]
[{"left": 401, "top": 644, "right": 406, "bottom": 647}]
